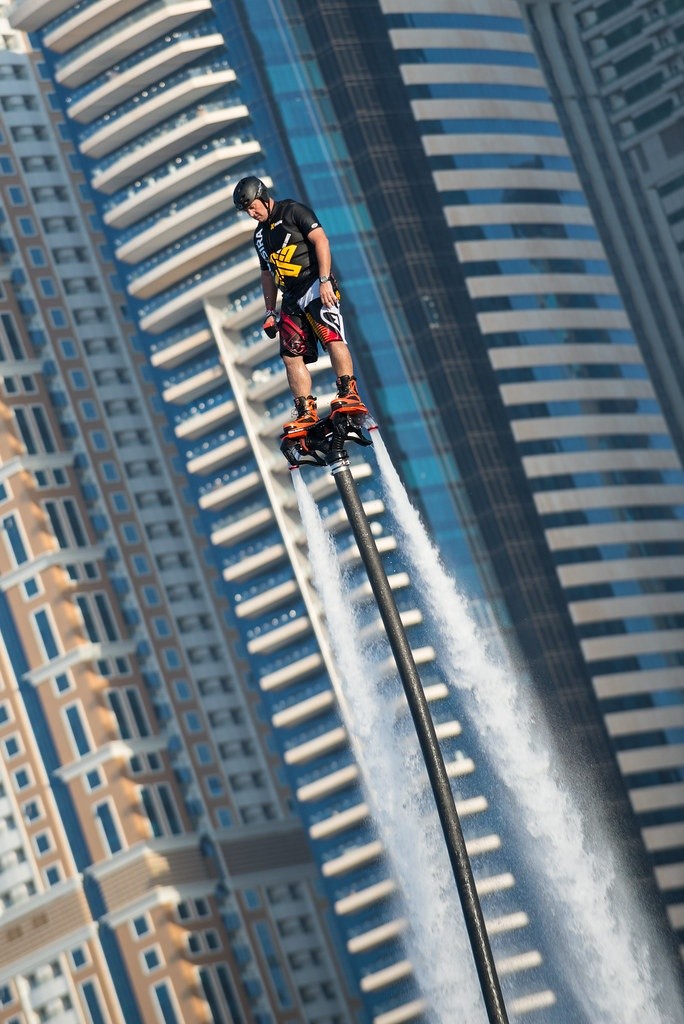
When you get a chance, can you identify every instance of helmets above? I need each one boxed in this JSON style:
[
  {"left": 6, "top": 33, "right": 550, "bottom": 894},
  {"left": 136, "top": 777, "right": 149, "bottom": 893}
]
[{"left": 233, "top": 175, "right": 269, "bottom": 211}]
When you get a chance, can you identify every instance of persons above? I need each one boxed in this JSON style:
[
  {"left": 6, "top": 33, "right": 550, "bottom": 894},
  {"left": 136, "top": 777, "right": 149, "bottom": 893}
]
[{"left": 233, "top": 177, "right": 361, "bottom": 432}]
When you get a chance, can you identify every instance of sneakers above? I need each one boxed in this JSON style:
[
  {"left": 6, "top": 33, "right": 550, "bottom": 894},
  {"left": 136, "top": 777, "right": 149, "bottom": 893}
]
[
  {"left": 283, "top": 395, "right": 320, "bottom": 433},
  {"left": 331, "top": 375, "right": 361, "bottom": 411}
]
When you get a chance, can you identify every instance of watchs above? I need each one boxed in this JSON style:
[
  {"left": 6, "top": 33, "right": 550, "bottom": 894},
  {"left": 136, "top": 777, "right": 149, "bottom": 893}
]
[{"left": 320, "top": 275, "right": 331, "bottom": 283}]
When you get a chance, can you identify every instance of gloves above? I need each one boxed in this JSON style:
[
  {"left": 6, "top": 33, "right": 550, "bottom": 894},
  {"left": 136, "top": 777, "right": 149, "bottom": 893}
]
[{"left": 264, "top": 305, "right": 279, "bottom": 339}]
